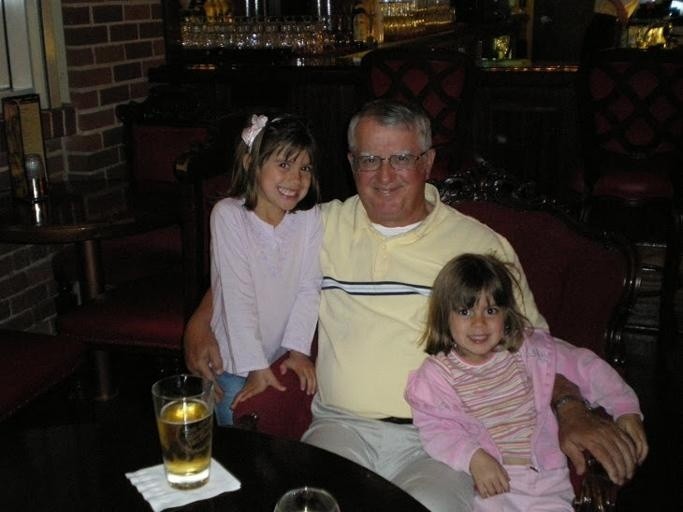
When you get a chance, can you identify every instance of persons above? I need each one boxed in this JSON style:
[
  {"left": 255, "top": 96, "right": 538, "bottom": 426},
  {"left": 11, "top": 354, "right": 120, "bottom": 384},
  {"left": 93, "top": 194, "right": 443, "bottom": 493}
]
[
  {"left": 181, "top": 99, "right": 637, "bottom": 512},
  {"left": 206, "top": 110, "right": 317, "bottom": 428},
  {"left": 405, "top": 254, "right": 649, "bottom": 512}
]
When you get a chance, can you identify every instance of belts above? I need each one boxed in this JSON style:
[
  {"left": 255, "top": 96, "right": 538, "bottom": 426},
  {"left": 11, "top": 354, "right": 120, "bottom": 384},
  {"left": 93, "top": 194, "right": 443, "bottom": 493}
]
[{"left": 381, "top": 417, "right": 411, "bottom": 424}]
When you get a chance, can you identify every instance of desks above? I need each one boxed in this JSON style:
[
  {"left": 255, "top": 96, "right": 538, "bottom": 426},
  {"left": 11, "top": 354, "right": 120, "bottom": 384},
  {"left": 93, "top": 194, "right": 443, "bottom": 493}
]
[
  {"left": 5, "top": 177, "right": 203, "bottom": 405},
  {"left": 3, "top": 424, "right": 432, "bottom": 507}
]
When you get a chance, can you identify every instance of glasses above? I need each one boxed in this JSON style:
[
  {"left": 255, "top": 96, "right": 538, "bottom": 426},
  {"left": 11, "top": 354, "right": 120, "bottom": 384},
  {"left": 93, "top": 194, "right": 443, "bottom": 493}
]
[{"left": 350, "top": 148, "right": 431, "bottom": 173}]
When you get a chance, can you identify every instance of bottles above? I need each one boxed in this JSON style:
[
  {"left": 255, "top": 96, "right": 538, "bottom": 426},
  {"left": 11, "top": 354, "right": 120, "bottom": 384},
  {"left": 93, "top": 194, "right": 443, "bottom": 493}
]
[{"left": 181, "top": 15, "right": 352, "bottom": 51}]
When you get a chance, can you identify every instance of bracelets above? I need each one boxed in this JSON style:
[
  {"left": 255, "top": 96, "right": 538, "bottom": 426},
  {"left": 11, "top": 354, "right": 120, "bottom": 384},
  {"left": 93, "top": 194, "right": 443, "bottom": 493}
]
[{"left": 552, "top": 393, "right": 594, "bottom": 415}]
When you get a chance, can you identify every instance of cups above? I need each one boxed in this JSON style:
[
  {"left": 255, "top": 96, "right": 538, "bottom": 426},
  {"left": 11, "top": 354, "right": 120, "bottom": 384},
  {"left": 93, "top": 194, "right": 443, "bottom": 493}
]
[
  {"left": 150, "top": 372, "right": 218, "bottom": 491},
  {"left": 270, "top": 486, "right": 343, "bottom": 512}
]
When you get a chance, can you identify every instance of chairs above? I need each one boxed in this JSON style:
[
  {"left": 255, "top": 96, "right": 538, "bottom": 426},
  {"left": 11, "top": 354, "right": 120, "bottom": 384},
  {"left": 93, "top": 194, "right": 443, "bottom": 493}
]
[
  {"left": 358, "top": 48, "right": 475, "bottom": 196},
  {"left": 572, "top": 54, "right": 680, "bottom": 231},
  {"left": 423, "top": 163, "right": 647, "bottom": 508},
  {"left": 57, "top": 101, "right": 223, "bottom": 396},
  {"left": 4, "top": 329, "right": 90, "bottom": 494}
]
